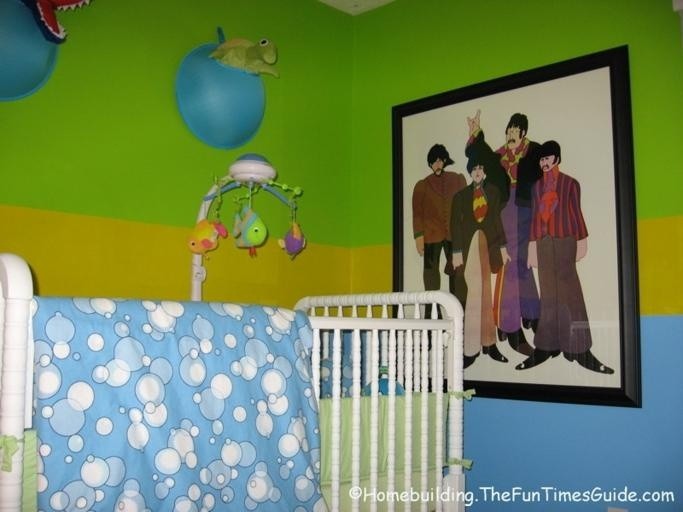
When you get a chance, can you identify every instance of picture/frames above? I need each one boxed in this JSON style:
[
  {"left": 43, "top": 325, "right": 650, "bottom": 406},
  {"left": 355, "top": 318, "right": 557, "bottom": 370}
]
[{"left": 391, "top": 43, "right": 643, "bottom": 410}]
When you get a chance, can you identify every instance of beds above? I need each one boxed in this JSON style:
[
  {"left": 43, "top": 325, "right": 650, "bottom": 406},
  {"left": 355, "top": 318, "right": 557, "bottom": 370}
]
[{"left": 1, "top": 251, "right": 466, "bottom": 511}]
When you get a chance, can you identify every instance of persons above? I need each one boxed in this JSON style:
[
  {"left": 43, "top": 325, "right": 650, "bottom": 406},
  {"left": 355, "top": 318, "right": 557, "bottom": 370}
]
[{"left": 409, "top": 107, "right": 614, "bottom": 377}]
